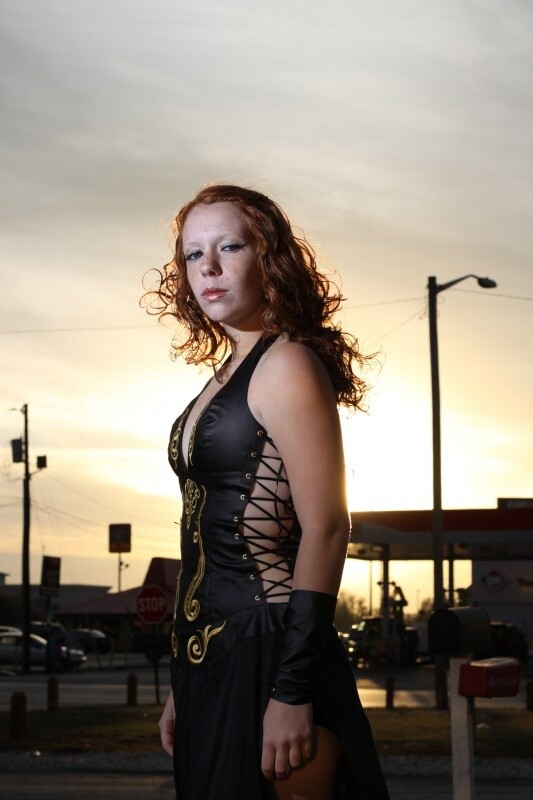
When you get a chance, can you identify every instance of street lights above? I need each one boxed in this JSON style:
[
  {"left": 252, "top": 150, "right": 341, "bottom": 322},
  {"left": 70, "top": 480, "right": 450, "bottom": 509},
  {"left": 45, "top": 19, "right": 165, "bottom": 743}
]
[
  {"left": 10, "top": 404, "right": 47, "bottom": 677},
  {"left": 427, "top": 274, "right": 498, "bottom": 608}
]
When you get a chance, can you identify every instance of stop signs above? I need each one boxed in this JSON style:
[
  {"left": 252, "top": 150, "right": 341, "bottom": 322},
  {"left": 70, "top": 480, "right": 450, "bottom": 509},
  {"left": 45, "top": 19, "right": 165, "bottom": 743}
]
[{"left": 136, "top": 585, "right": 169, "bottom": 625}]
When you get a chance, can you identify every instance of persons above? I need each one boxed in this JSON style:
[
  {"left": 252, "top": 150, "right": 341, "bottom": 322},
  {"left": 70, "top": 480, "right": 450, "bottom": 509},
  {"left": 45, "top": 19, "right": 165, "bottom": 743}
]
[{"left": 140, "top": 186, "right": 388, "bottom": 800}]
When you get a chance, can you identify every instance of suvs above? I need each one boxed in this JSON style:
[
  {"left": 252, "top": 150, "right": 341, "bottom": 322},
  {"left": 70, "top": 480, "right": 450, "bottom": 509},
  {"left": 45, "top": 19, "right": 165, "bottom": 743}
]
[{"left": 17, "top": 620, "right": 66, "bottom": 647}]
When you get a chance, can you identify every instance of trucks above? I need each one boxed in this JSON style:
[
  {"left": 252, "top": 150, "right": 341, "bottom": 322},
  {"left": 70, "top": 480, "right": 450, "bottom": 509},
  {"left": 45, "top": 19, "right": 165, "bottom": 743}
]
[{"left": 345, "top": 618, "right": 421, "bottom": 669}]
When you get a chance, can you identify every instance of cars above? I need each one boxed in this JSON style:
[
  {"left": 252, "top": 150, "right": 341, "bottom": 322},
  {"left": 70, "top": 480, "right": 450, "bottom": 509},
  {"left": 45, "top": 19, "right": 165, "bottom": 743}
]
[
  {"left": 0, "top": 625, "right": 88, "bottom": 675},
  {"left": 68, "top": 628, "right": 113, "bottom": 655}
]
